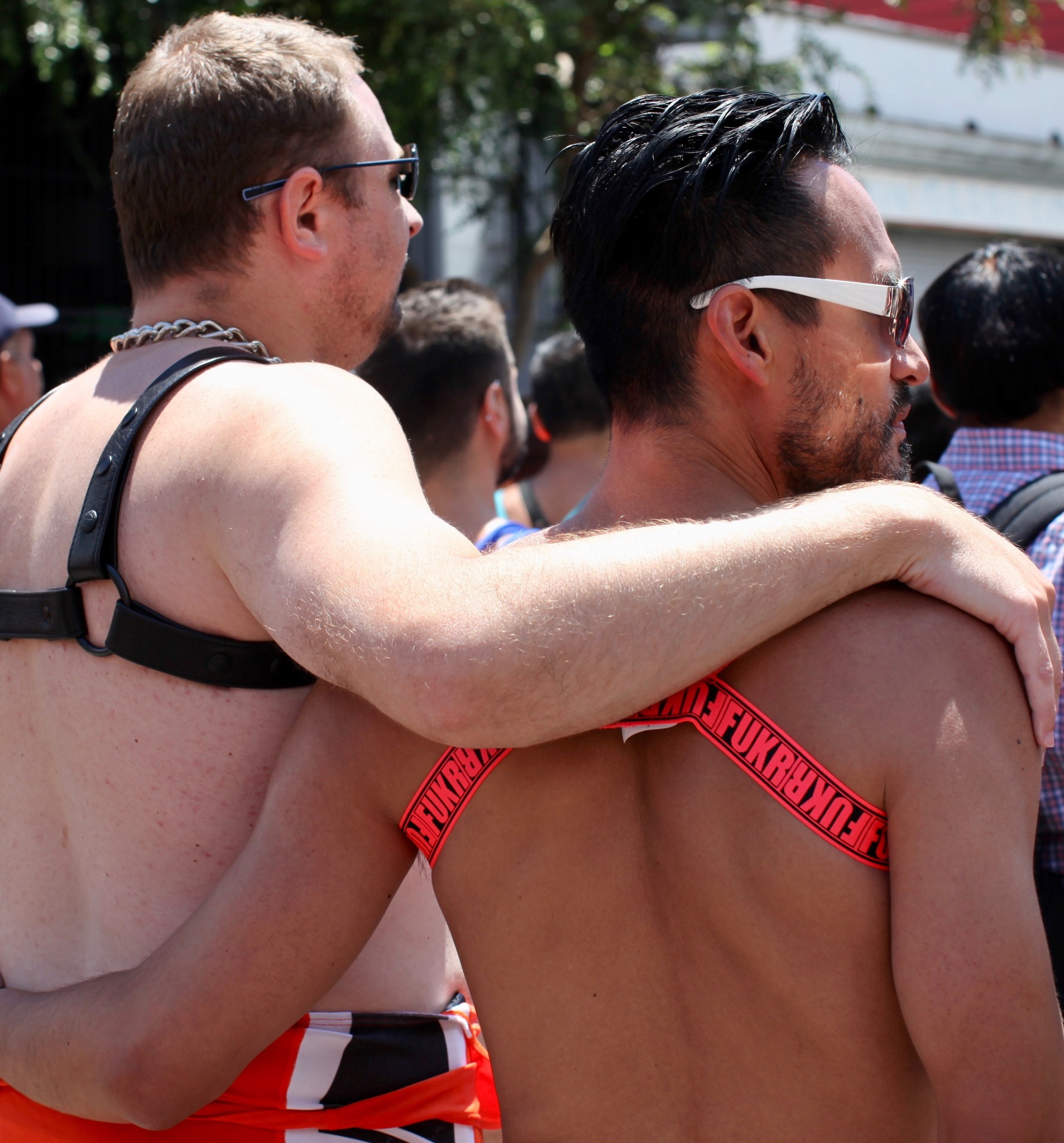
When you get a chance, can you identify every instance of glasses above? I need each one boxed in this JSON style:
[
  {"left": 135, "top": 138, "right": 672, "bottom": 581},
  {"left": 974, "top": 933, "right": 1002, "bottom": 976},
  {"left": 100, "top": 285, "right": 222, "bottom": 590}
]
[
  {"left": 241, "top": 143, "right": 421, "bottom": 203},
  {"left": 689, "top": 275, "right": 914, "bottom": 347}
]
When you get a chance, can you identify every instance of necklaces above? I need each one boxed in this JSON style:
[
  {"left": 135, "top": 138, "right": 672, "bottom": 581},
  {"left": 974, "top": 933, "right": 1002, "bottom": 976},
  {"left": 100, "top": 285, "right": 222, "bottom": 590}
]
[{"left": 108, "top": 318, "right": 279, "bottom": 366}]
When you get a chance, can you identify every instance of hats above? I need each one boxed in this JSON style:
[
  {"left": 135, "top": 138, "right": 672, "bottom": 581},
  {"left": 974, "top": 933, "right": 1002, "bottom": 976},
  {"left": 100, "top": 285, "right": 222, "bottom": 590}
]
[{"left": 0, "top": 292, "right": 60, "bottom": 340}]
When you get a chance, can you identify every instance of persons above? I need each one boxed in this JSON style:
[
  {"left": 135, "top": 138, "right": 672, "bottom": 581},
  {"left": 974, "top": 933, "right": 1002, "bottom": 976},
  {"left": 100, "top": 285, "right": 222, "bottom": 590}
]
[{"left": 0, "top": 10, "right": 1063, "bottom": 1142}]
